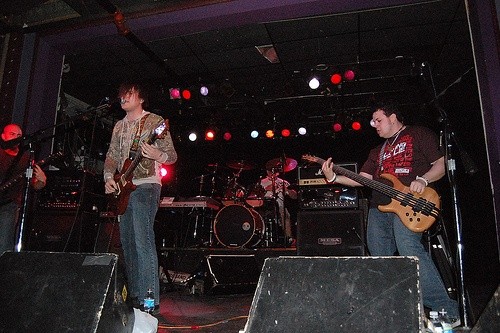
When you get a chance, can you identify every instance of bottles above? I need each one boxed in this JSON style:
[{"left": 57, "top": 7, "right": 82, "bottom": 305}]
[
  {"left": 144, "top": 289, "right": 155, "bottom": 316},
  {"left": 438, "top": 309, "right": 452, "bottom": 333},
  {"left": 422, "top": 311, "right": 444, "bottom": 333}
]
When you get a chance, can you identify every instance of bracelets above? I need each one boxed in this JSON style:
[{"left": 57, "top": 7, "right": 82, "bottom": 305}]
[
  {"left": 327, "top": 172, "right": 336, "bottom": 183},
  {"left": 416, "top": 176, "right": 428, "bottom": 186}
]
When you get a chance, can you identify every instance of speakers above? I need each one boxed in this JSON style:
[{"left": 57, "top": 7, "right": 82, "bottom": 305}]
[
  {"left": 153, "top": 200, "right": 221, "bottom": 248},
  {"left": 469, "top": 286, "right": 500, "bottom": 333},
  {"left": 297, "top": 208, "right": 365, "bottom": 257},
  {"left": 183, "top": 254, "right": 262, "bottom": 296},
  {"left": 30, "top": 209, "right": 98, "bottom": 254},
  {"left": 94, "top": 211, "right": 124, "bottom": 254},
  {"left": 0, "top": 249, "right": 135, "bottom": 333},
  {"left": 244, "top": 255, "right": 428, "bottom": 333}
]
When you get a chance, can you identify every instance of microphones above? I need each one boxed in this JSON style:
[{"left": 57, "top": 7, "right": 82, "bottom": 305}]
[{"left": 104, "top": 96, "right": 126, "bottom": 104}]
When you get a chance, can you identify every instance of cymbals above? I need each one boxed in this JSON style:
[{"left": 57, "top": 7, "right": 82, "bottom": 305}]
[
  {"left": 227, "top": 161, "right": 256, "bottom": 170},
  {"left": 266, "top": 158, "right": 297, "bottom": 173}
]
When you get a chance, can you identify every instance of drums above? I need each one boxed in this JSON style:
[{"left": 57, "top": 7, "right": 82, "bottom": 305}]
[
  {"left": 213, "top": 203, "right": 266, "bottom": 249},
  {"left": 221, "top": 183, "right": 247, "bottom": 206},
  {"left": 247, "top": 185, "right": 266, "bottom": 207}
]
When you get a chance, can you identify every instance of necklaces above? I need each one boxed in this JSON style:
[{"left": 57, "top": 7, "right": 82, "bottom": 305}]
[
  {"left": 392, "top": 125, "right": 407, "bottom": 137},
  {"left": 386, "top": 132, "right": 400, "bottom": 151}
]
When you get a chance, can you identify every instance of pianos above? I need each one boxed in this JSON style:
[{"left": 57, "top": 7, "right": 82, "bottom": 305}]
[{"left": 159, "top": 194, "right": 220, "bottom": 247}]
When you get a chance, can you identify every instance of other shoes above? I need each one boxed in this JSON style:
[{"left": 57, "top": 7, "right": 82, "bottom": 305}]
[
  {"left": 127, "top": 299, "right": 140, "bottom": 312},
  {"left": 424, "top": 316, "right": 460, "bottom": 331},
  {"left": 287, "top": 238, "right": 296, "bottom": 247},
  {"left": 137, "top": 304, "right": 160, "bottom": 316}
]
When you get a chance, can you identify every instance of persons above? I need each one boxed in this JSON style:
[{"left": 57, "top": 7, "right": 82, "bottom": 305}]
[
  {"left": 255, "top": 171, "right": 297, "bottom": 247},
  {"left": 104, "top": 80, "right": 178, "bottom": 315},
  {"left": 0, "top": 124, "right": 46, "bottom": 255},
  {"left": 322, "top": 104, "right": 461, "bottom": 328}
]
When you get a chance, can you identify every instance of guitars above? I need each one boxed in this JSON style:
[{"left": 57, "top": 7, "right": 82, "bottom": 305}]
[
  {"left": 0, "top": 149, "right": 65, "bottom": 196},
  {"left": 108, "top": 117, "right": 170, "bottom": 215},
  {"left": 301, "top": 152, "right": 441, "bottom": 233}
]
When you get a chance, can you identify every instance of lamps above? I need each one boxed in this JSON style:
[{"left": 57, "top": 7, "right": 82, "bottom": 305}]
[{"left": 168, "top": 71, "right": 368, "bottom": 145}]
